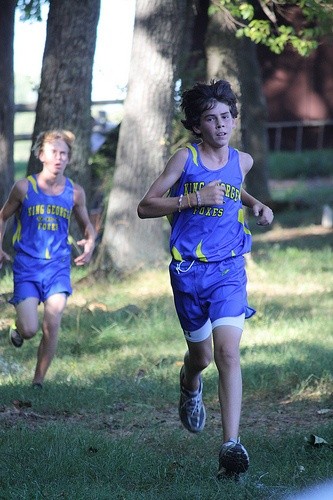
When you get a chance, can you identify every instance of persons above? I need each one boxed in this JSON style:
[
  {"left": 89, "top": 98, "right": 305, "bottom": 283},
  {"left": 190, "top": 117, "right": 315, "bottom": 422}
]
[
  {"left": 95, "top": 110, "right": 108, "bottom": 133},
  {"left": 137, "top": 77, "right": 276, "bottom": 486},
  {"left": 0, "top": 126, "right": 98, "bottom": 394}
]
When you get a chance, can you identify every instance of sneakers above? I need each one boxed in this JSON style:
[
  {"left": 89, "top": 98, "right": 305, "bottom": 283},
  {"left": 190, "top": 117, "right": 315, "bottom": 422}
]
[
  {"left": 217, "top": 438, "right": 251, "bottom": 481},
  {"left": 179, "top": 365, "right": 207, "bottom": 433},
  {"left": 33, "top": 383, "right": 43, "bottom": 389},
  {"left": 9, "top": 309, "right": 24, "bottom": 349}
]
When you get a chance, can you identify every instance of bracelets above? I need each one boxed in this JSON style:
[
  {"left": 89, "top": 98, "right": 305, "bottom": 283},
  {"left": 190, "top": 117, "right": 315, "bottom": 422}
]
[
  {"left": 176, "top": 194, "right": 185, "bottom": 213},
  {"left": 194, "top": 191, "right": 201, "bottom": 208},
  {"left": 186, "top": 194, "right": 192, "bottom": 208}
]
